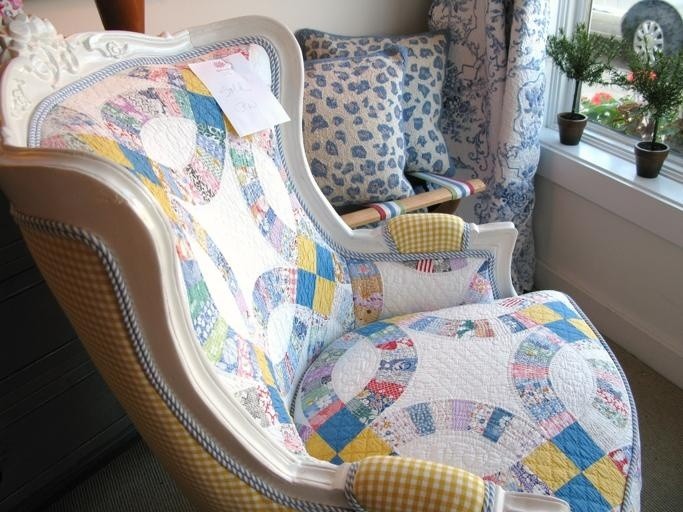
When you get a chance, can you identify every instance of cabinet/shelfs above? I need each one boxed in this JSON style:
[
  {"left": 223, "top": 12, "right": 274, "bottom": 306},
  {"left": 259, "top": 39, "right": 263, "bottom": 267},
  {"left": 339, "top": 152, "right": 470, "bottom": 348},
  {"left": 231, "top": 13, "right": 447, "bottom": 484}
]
[{"left": 1, "top": 184, "right": 144, "bottom": 512}]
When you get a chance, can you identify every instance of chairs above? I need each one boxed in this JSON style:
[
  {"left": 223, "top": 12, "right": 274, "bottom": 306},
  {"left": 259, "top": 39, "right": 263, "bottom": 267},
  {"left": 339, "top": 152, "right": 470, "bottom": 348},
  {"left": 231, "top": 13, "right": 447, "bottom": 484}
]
[{"left": 1, "top": 6, "right": 643, "bottom": 512}]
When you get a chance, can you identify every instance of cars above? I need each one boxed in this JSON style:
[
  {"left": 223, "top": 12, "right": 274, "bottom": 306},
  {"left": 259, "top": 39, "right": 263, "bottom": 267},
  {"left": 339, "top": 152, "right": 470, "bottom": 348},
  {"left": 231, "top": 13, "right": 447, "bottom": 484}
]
[{"left": 588, "top": 0, "right": 682, "bottom": 75}]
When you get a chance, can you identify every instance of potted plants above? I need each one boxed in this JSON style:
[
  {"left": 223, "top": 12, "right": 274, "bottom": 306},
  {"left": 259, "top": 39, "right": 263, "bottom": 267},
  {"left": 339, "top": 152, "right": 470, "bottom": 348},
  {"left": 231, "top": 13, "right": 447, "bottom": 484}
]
[
  {"left": 624, "top": 42, "right": 683, "bottom": 178},
  {"left": 544, "top": 20, "right": 629, "bottom": 146}
]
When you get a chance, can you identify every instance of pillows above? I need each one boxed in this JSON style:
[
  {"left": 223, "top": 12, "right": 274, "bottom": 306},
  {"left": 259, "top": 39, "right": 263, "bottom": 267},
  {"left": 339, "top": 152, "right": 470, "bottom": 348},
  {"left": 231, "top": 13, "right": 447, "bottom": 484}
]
[
  {"left": 299, "top": 44, "right": 416, "bottom": 208},
  {"left": 294, "top": 28, "right": 455, "bottom": 177}
]
